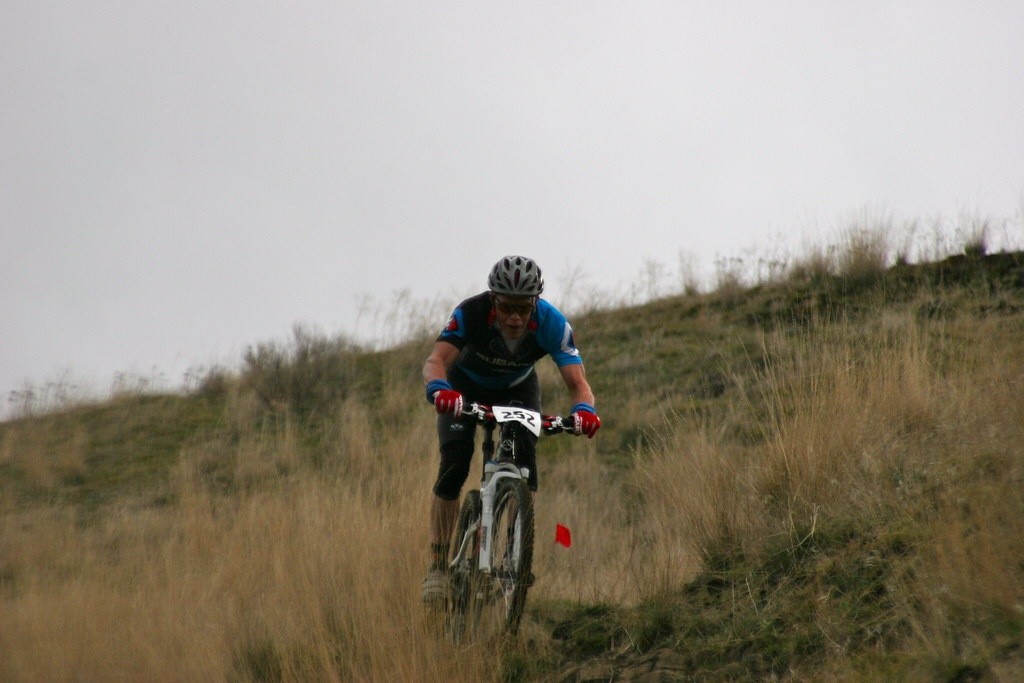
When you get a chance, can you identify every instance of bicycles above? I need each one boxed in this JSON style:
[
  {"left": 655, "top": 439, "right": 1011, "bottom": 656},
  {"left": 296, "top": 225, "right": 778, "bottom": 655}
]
[{"left": 421, "top": 391, "right": 593, "bottom": 644}]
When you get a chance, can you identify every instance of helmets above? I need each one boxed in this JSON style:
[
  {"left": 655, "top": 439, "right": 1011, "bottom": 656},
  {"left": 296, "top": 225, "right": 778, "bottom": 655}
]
[{"left": 487, "top": 255, "right": 545, "bottom": 296}]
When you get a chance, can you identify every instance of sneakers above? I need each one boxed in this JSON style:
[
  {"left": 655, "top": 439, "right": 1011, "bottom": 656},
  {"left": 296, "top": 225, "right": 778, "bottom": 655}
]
[
  {"left": 420, "top": 568, "right": 450, "bottom": 605},
  {"left": 503, "top": 552, "right": 535, "bottom": 588}
]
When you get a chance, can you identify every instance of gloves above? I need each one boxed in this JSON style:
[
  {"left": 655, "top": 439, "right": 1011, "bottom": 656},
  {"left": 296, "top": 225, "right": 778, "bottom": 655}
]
[
  {"left": 569, "top": 402, "right": 601, "bottom": 438},
  {"left": 425, "top": 379, "right": 463, "bottom": 419}
]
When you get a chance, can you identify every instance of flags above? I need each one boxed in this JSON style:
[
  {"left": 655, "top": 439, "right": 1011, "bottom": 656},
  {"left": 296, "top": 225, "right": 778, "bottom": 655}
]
[{"left": 555, "top": 523, "right": 572, "bottom": 548}]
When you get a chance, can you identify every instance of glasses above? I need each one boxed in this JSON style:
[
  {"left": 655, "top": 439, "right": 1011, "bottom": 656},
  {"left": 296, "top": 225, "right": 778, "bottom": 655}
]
[{"left": 494, "top": 296, "right": 535, "bottom": 315}]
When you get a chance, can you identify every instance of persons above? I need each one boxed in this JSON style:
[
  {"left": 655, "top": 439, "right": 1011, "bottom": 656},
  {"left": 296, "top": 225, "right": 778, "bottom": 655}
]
[{"left": 423, "top": 255, "right": 601, "bottom": 610}]
